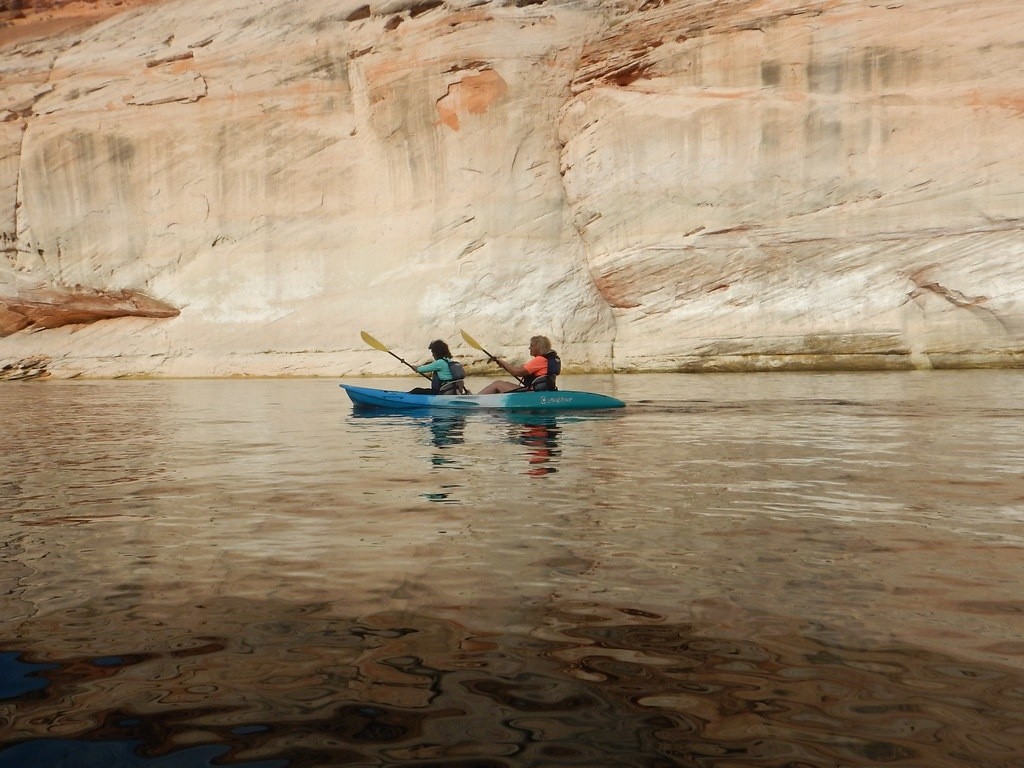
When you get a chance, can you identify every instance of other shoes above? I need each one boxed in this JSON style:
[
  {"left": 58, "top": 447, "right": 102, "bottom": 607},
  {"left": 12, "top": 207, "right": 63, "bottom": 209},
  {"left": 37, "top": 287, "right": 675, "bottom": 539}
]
[
  {"left": 456, "top": 389, "right": 462, "bottom": 395},
  {"left": 468, "top": 389, "right": 472, "bottom": 394}
]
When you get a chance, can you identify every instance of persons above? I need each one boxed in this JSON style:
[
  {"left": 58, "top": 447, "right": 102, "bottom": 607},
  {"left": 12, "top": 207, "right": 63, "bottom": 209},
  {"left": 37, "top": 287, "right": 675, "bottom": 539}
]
[
  {"left": 477, "top": 335, "right": 552, "bottom": 395},
  {"left": 408, "top": 340, "right": 454, "bottom": 395}
]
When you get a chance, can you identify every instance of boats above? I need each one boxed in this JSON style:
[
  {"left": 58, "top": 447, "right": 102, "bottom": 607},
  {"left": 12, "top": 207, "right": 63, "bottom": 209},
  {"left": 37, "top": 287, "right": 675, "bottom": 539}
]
[{"left": 340, "top": 380, "right": 627, "bottom": 413}]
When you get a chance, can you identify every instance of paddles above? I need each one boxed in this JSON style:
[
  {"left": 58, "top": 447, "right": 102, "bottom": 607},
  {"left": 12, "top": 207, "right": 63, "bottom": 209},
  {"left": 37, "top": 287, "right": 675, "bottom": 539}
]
[
  {"left": 359, "top": 330, "right": 433, "bottom": 382},
  {"left": 459, "top": 328, "right": 524, "bottom": 385}
]
